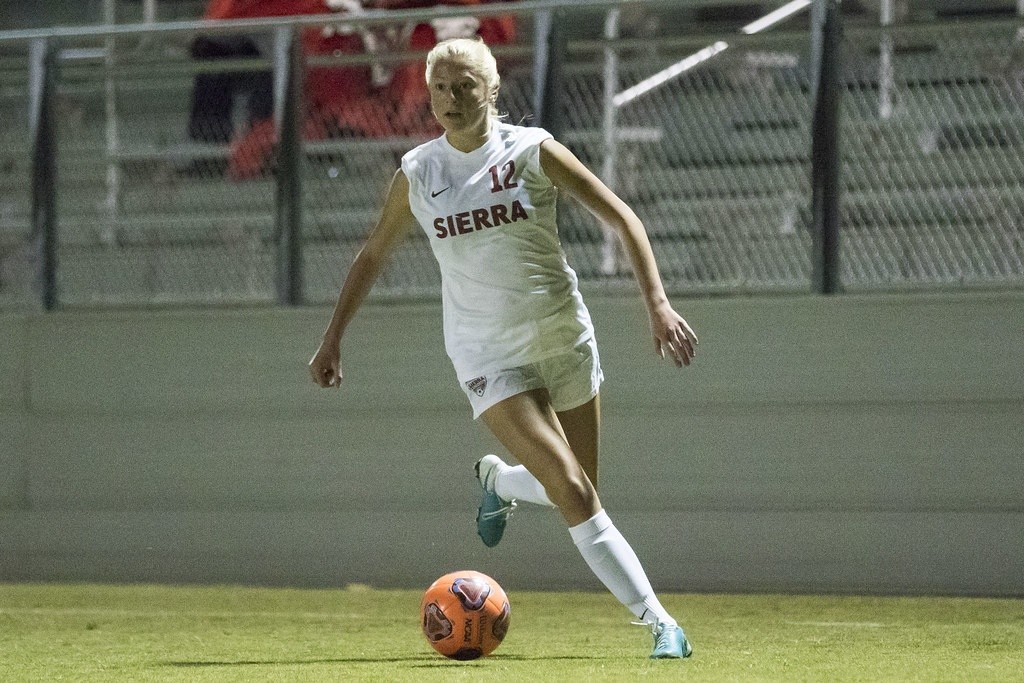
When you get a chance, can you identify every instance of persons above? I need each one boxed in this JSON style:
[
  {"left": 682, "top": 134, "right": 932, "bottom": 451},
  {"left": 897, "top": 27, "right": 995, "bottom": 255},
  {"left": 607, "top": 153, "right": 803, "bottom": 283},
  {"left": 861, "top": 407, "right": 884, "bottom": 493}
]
[
  {"left": 174, "top": 0, "right": 515, "bottom": 178},
  {"left": 306, "top": 38, "right": 701, "bottom": 659}
]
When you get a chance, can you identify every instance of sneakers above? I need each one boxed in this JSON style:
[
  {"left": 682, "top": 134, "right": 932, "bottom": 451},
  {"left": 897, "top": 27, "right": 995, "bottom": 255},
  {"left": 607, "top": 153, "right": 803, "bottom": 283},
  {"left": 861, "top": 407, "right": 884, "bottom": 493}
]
[
  {"left": 474, "top": 454, "right": 517, "bottom": 548},
  {"left": 648, "top": 617, "right": 693, "bottom": 658}
]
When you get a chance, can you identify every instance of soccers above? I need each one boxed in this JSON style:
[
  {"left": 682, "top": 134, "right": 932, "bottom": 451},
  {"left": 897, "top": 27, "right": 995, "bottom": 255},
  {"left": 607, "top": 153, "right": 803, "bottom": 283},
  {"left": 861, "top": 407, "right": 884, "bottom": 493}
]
[{"left": 418, "top": 569, "right": 513, "bottom": 661}]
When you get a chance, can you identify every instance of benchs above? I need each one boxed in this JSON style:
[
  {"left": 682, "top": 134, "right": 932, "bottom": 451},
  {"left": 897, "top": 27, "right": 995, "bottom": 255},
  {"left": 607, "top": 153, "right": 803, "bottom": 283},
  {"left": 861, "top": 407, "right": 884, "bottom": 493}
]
[{"left": 0, "top": 0, "right": 1024, "bottom": 296}]
